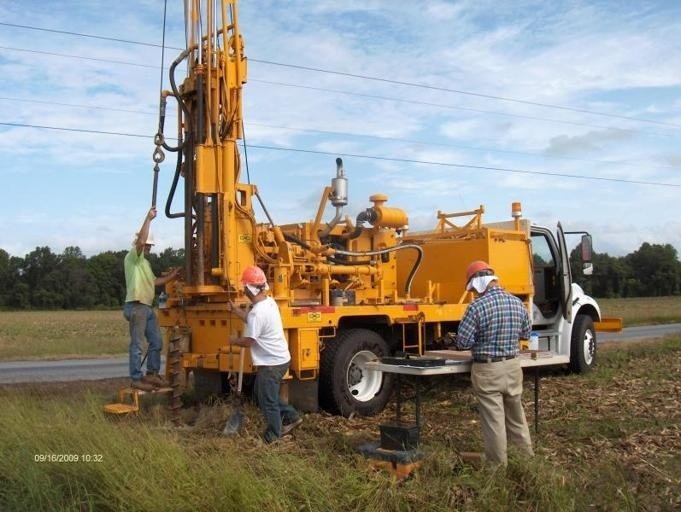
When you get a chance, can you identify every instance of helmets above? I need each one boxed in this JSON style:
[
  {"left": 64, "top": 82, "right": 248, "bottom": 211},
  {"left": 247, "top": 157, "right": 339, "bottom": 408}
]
[
  {"left": 242, "top": 266, "right": 266, "bottom": 286},
  {"left": 464, "top": 261, "right": 494, "bottom": 290},
  {"left": 134, "top": 231, "right": 154, "bottom": 245}
]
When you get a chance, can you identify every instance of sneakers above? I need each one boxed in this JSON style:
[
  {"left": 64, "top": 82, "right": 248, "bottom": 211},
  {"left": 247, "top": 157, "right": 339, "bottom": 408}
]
[
  {"left": 132, "top": 375, "right": 168, "bottom": 390},
  {"left": 282, "top": 418, "right": 302, "bottom": 434}
]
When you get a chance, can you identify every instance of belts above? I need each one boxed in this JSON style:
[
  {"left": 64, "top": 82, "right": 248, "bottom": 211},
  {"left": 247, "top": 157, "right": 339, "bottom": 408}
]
[{"left": 474, "top": 355, "right": 515, "bottom": 363}]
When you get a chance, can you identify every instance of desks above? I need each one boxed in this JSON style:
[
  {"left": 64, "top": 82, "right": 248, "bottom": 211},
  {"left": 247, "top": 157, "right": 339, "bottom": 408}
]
[{"left": 366, "top": 351, "right": 552, "bottom": 450}]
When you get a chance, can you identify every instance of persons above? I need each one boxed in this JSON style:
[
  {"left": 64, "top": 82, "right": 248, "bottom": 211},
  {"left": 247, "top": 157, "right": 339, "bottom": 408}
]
[
  {"left": 124, "top": 206, "right": 184, "bottom": 392},
  {"left": 225, "top": 263, "right": 305, "bottom": 446},
  {"left": 458, "top": 260, "right": 544, "bottom": 463}
]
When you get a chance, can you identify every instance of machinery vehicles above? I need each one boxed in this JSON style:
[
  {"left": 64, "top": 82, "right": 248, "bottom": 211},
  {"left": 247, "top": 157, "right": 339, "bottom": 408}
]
[{"left": 105, "top": 0, "right": 623, "bottom": 423}]
